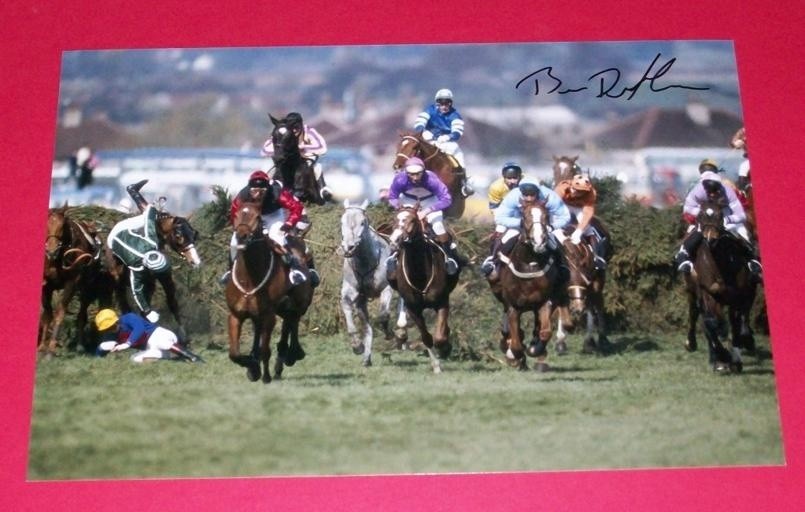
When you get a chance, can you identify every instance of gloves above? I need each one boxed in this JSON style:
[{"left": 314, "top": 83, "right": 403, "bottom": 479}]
[
  {"left": 280, "top": 223, "right": 292, "bottom": 235},
  {"left": 146, "top": 311, "right": 160, "bottom": 323},
  {"left": 571, "top": 228, "right": 583, "bottom": 244},
  {"left": 101, "top": 339, "right": 130, "bottom": 353}
]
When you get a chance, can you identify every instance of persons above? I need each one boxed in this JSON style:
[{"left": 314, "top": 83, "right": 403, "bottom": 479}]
[
  {"left": 386, "top": 158, "right": 457, "bottom": 276},
  {"left": 260, "top": 114, "right": 332, "bottom": 201},
  {"left": 730, "top": 127, "right": 750, "bottom": 198},
  {"left": 222, "top": 172, "right": 306, "bottom": 284},
  {"left": 554, "top": 173, "right": 605, "bottom": 269},
  {"left": 106, "top": 180, "right": 171, "bottom": 323},
  {"left": 414, "top": 89, "right": 474, "bottom": 198},
  {"left": 700, "top": 159, "right": 750, "bottom": 209},
  {"left": 95, "top": 308, "right": 202, "bottom": 363},
  {"left": 676, "top": 171, "right": 762, "bottom": 274},
  {"left": 481, "top": 175, "right": 570, "bottom": 281},
  {"left": 59, "top": 146, "right": 100, "bottom": 205},
  {"left": 488, "top": 163, "right": 537, "bottom": 219}
]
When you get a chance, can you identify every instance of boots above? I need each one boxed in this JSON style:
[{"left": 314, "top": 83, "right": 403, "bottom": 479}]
[{"left": 159, "top": 343, "right": 201, "bottom": 364}]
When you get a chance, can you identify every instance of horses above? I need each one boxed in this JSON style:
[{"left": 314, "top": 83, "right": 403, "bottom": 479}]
[
  {"left": 339, "top": 196, "right": 408, "bottom": 367},
  {"left": 224, "top": 189, "right": 316, "bottom": 385},
  {"left": 36, "top": 197, "right": 203, "bottom": 354},
  {"left": 393, "top": 197, "right": 461, "bottom": 375},
  {"left": 482, "top": 154, "right": 609, "bottom": 373},
  {"left": 266, "top": 112, "right": 326, "bottom": 206},
  {"left": 680, "top": 170, "right": 768, "bottom": 377},
  {"left": 735, "top": 362, "right": 736, "bottom": 363},
  {"left": 392, "top": 128, "right": 466, "bottom": 221}
]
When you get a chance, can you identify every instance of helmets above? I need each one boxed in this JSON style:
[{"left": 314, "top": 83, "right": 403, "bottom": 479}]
[
  {"left": 700, "top": 159, "right": 721, "bottom": 172},
  {"left": 569, "top": 174, "right": 593, "bottom": 193},
  {"left": 434, "top": 88, "right": 453, "bottom": 108},
  {"left": 250, "top": 171, "right": 270, "bottom": 188},
  {"left": 518, "top": 175, "right": 540, "bottom": 194},
  {"left": 145, "top": 251, "right": 171, "bottom": 273},
  {"left": 95, "top": 308, "right": 119, "bottom": 330},
  {"left": 503, "top": 162, "right": 520, "bottom": 178},
  {"left": 702, "top": 173, "right": 721, "bottom": 192},
  {"left": 404, "top": 157, "right": 426, "bottom": 174}
]
